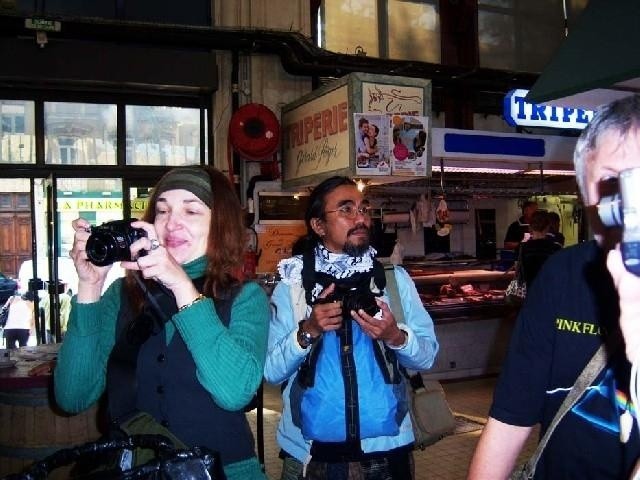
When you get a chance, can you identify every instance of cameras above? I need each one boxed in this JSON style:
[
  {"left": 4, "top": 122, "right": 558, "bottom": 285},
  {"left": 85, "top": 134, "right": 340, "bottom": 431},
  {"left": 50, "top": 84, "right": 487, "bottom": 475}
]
[
  {"left": 597, "top": 168, "right": 640, "bottom": 277},
  {"left": 85, "top": 218, "right": 148, "bottom": 266},
  {"left": 334, "top": 272, "right": 380, "bottom": 319}
]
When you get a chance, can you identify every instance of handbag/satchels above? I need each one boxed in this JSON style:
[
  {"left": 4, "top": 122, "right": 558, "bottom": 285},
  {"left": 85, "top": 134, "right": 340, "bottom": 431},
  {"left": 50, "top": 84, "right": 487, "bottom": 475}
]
[{"left": 410, "top": 381, "right": 456, "bottom": 451}]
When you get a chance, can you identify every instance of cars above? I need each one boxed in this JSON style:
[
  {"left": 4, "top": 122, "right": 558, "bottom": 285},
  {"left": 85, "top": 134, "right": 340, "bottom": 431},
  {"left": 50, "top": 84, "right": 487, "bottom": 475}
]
[{"left": 0, "top": 271, "right": 20, "bottom": 306}]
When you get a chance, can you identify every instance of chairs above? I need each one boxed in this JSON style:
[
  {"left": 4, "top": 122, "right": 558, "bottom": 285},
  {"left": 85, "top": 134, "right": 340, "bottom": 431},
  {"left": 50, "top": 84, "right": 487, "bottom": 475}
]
[{"left": 240, "top": 374, "right": 266, "bottom": 473}]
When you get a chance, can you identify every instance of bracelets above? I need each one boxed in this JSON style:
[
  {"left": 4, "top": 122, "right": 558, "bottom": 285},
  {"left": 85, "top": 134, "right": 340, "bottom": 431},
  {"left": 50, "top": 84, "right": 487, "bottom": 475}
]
[{"left": 173, "top": 295, "right": 209, "bottom": 312}]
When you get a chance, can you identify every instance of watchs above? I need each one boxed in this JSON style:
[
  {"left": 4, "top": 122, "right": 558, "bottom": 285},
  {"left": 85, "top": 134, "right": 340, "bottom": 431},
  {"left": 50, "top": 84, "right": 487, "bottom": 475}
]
[{"left": 297, "top": 325, "right": 313, "bottom": 343}]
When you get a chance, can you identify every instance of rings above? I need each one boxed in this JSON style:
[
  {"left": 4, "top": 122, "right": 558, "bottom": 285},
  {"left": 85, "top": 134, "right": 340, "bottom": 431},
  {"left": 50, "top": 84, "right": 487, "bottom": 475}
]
[{"left": 149, "top": 239, "right": 161, "bottom": 250}]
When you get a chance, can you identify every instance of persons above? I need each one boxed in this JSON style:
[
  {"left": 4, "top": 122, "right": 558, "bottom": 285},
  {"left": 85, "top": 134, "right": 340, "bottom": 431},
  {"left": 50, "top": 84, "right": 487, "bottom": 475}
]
[
  {"left": 604, "top": 240, "right": 640, "bottom": 370},
  {"left": 469, "top": 91, "right": 640, "bottom": 479},
  {"left": 43, "top": 284, "right": 72, "bottom": 344},
  {"left": 50, "top": 164, "right": 269, "bottom": 477},
  {"left": 261, "top": 178, "right": 439, "bottom": 480},
  {"left": 356, "top": 116, "right": 378, "bottom": 159},
  {"left": 503, "top": 197, "right": 565, "bottom": 296},
  {"left": 0, "top": 288, "right": 37, "bottom": 347}
]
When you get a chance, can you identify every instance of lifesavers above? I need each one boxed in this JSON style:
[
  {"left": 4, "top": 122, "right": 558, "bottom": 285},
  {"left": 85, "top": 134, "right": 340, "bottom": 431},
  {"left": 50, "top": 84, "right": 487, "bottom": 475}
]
[{"left": 230, "top": 102, "right": 280, "bottom": 158}]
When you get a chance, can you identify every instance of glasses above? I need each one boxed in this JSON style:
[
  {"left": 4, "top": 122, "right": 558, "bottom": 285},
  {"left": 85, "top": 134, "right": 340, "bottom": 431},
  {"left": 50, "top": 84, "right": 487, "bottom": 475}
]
[{"left": 326, "top": 202, "right": 371, "bottom": 219}]
